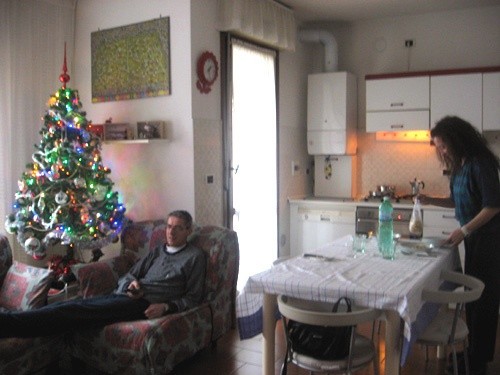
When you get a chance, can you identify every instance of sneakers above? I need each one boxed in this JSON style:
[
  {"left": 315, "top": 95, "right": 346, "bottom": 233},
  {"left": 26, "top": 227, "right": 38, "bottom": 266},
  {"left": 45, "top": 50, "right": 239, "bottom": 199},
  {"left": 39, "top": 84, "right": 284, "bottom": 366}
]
[
  {"left": 446, "top": 365, "right": 490, "bottom": 375},
  {"left": 449, "top": 350, "right": 494, "bottom": 362}
]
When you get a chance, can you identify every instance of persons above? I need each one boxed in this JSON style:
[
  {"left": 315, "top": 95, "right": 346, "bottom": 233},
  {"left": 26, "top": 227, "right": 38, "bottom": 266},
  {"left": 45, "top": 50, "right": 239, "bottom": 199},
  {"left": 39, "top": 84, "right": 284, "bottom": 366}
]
[
  {"left": 0, "top": 209, "right": 206, "bottom": 339},
  {"left": 430, "top": 116, "right": 499, "bottom": 375}
]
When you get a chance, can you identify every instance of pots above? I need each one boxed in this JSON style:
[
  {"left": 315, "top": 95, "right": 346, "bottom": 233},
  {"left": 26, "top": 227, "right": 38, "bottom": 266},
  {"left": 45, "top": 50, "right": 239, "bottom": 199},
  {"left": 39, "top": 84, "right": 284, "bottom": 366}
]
[{"left": 370, "top": 190, "right": 390, "bottom": 196}]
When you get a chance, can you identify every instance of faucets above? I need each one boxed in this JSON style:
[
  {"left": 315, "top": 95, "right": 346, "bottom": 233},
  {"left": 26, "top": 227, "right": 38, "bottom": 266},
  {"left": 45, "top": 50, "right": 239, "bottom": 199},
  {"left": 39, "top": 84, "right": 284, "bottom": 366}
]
[{"left": 410, "top": 175, "right": 427, "bottom": 196}]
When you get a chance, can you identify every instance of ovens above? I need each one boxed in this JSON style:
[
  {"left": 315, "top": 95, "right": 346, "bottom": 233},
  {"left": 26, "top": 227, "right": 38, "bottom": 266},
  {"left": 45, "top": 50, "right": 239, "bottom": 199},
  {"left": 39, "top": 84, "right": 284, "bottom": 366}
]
[{"left": 356, "top": 205, "right": 423, "bottom": 239}]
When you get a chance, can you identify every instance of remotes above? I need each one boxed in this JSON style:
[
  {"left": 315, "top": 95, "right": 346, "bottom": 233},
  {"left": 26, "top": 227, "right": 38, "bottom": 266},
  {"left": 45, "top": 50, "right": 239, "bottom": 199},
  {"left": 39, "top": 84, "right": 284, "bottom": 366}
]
[{"left": 121, "top": 288, "right": 145, "bottom": 297}]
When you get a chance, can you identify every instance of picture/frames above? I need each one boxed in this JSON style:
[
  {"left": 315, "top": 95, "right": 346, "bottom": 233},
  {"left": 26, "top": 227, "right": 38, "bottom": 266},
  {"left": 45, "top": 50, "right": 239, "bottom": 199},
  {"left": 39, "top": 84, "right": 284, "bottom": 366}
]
[{"left": 90, "top": 15, "right": 171, "bottom": 102}]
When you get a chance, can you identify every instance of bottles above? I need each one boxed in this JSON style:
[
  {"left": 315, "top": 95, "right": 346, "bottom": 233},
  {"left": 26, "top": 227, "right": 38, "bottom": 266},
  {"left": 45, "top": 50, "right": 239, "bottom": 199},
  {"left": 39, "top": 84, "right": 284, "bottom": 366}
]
[
  {"left": 377, "top": 196, "right": 393, "bottom": 253},
  {"left": 409, "top": 197, "right": 423, "bottom": 239}
]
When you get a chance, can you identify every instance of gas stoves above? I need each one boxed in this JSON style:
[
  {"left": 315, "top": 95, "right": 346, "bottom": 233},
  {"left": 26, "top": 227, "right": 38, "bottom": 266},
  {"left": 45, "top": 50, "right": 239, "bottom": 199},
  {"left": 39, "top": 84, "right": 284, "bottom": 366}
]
[{"left": 362, "top": 193, "right": 424, "bottom": 203}]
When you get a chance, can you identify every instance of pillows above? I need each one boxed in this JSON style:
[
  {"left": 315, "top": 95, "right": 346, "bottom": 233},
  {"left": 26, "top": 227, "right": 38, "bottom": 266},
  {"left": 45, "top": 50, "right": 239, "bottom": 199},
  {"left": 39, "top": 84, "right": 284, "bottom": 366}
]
[{"left": 0, "top": 261, "right": 55, "bottom": 313}]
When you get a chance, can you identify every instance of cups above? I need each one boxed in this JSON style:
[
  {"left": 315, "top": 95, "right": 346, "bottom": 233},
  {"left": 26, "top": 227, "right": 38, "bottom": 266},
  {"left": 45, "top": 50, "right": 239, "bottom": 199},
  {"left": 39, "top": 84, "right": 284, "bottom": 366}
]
[
  {"left": 345, "top": 241, "right": 358, "bottom": 257},
  {"left": 381, "top": 234, "right": 400, "bottom": 260},
  {"left": 350, "top": 234, "right": 367, "bottom": 253}
]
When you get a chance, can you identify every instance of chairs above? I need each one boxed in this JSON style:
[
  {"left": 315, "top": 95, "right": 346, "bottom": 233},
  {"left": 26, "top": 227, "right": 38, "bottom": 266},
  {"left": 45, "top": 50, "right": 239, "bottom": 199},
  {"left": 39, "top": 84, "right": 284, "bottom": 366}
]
[
  {"left": 278, "top": 294, "right": 382, "bottom": 375},
  {"left": 414, "top": 269, "right": 486, "bottom": 375}
]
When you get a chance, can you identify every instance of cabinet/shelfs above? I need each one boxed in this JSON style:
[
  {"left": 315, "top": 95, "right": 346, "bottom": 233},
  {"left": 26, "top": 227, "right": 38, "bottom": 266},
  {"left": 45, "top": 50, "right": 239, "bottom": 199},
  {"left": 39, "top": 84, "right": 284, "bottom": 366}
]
[
  {"left": 365, "top": 74, "right": 431, "bottom": 132},
  {"left": 429, "top": 71, "right": 482, "bottom": 133},
  {"left": 307, "top": 71, "right": 358, "bottom": 156},
  {"left": 483, "top": 69, "right": 500, "bottom": 131},
  {"left": 422, "top": 210, "right": 466, "bottom": 262}
]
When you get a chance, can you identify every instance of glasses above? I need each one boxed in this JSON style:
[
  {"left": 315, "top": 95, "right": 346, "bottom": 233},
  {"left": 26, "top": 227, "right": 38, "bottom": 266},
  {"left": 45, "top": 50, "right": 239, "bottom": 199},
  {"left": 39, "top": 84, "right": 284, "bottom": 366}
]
[{"left": 166, "top": 225, "right": 184, "bottom": 231}]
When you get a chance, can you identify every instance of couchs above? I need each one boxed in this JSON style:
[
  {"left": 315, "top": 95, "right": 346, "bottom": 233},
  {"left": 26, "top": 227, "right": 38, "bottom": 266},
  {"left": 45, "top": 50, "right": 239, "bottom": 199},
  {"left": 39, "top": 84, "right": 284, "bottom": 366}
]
[
  {"left": 1, "top": 235, "right": 66, "bottom": 375},
  {"left": 74, "top": 218, "right": 240, "bottom": 374}
]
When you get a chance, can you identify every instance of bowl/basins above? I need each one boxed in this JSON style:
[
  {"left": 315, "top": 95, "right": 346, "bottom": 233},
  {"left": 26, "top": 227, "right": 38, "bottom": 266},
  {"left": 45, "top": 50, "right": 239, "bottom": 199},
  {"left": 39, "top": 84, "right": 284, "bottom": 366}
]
[
  {"left": 415, "top": 243, "right": 434, "bottom": 255},
  {"left": 378, "top": 184, "right": 395, "bottom": 192}
]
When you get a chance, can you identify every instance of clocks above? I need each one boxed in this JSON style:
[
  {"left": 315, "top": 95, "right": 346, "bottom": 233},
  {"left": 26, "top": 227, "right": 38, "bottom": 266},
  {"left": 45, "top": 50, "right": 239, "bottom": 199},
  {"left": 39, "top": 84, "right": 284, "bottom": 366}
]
[{"left": 196, "top": 51, "right": 219, "bottom": 94}]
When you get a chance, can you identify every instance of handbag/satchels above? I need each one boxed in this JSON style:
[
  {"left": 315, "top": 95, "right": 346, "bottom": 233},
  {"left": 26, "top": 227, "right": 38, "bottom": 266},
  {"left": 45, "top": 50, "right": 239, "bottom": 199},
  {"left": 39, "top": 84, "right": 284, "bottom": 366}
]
[
  {"left": 48, "top": 282, "right": 83, "bottom": 303},
  {"left": 286, "top": 297, "right": 356, "bottom": 361}
]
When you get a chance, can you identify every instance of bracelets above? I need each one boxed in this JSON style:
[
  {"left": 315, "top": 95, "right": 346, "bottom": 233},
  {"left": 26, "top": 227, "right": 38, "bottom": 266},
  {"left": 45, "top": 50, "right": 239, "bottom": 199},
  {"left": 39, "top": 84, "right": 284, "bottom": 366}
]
[{"left": 459, "top": 226, "right": 470, "bottom": 238}]
